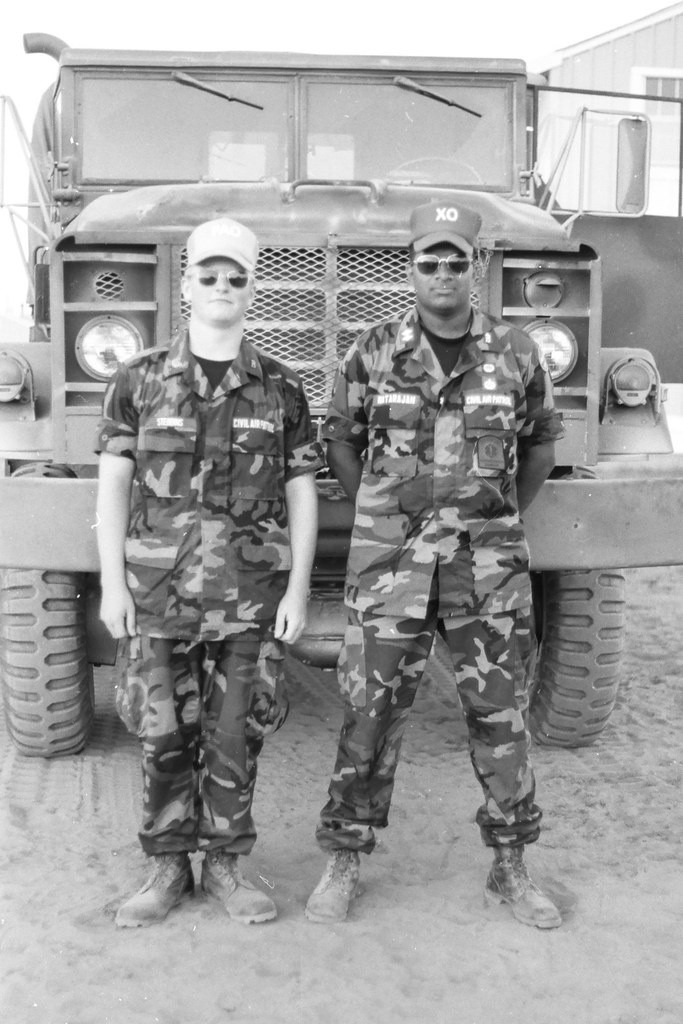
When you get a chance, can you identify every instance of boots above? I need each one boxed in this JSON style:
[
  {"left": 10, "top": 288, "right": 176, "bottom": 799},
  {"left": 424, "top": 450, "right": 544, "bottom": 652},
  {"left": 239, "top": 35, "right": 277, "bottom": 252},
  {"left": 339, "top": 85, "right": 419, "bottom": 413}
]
[
  {"left": 115, "top": 850, "right": 194, "bottom": 927},
  {"left": 484, "top": 844, "right": 562, "bottom": 928},
  {"left": 200, "top": 848, "right": 277, "bottom": 924},
  {"left": 305, "top": 849, "right": 361, "bottom": 924}
]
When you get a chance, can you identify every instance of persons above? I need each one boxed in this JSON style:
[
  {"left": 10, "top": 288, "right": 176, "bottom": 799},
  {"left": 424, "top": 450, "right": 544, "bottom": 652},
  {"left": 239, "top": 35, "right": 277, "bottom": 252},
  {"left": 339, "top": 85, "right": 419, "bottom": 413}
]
[
  {"left": 300, "top": 200, "right": 568, "bottom": 932},
  {"left": 92, "top": 216, "right": 320, "bottom": 931}
]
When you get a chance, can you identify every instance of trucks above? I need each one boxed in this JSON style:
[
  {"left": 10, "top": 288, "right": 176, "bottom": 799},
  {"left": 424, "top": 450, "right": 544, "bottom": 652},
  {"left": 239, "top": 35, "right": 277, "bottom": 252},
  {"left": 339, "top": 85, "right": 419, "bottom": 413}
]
[{"left": 0, "top": 23, "right": 680, "bottom": 760}]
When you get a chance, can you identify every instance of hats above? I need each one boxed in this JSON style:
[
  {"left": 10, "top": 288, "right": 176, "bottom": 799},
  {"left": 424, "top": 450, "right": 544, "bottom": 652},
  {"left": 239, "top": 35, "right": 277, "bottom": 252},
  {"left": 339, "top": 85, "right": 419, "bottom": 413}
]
[
  {"left": 186, "top": 217, "right": 259, "bottom": 273},
  {"left": 409, "top": 199, "right": 482, "bottom": 254}
]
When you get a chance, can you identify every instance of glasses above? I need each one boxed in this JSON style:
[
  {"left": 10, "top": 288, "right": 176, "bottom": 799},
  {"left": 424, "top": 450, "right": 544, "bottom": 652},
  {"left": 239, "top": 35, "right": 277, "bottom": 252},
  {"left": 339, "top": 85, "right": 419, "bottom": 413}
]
[
  {"left": 411, "top": 254, "right": 473, "bottom": 274},
  {"left": 187, "top": 269, "right": 253, "bottom": 288}
]
